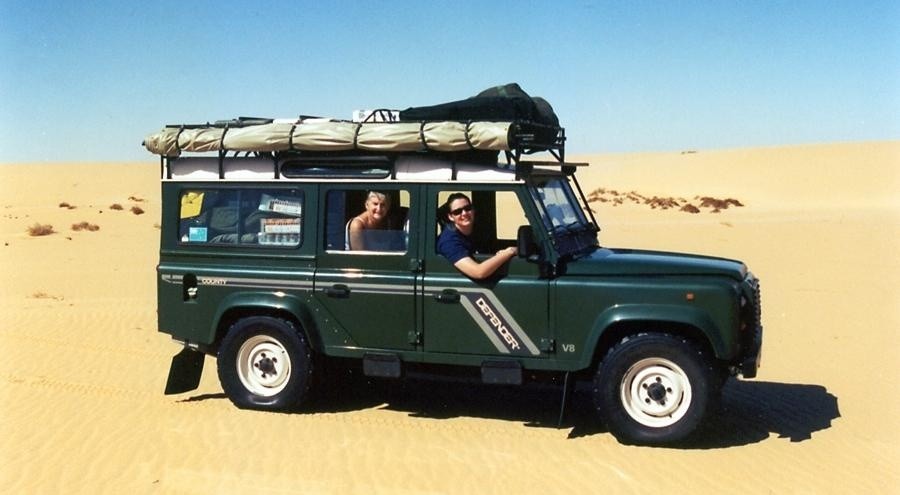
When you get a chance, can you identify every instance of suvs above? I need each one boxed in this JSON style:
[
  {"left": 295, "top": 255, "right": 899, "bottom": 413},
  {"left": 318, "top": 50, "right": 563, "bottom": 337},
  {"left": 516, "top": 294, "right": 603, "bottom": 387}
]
[{"left": 156, "top": 109, "right": 762, "bottom": 446}]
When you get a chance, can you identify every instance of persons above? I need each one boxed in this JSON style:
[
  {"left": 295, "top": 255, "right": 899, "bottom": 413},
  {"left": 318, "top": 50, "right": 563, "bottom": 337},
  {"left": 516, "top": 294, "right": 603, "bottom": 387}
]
[
  {"left": 437, "top": 193, "right": 518, "bottom": 279},
  {"left": 345, "top": 191, "right": 392, "bottom": 250}
]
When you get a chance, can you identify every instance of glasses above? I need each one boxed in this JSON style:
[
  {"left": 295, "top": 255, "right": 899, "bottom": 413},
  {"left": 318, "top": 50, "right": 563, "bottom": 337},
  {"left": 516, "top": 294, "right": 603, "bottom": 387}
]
[{"left": 450, "top": 204, "right": 472, "bottom": 216}]
